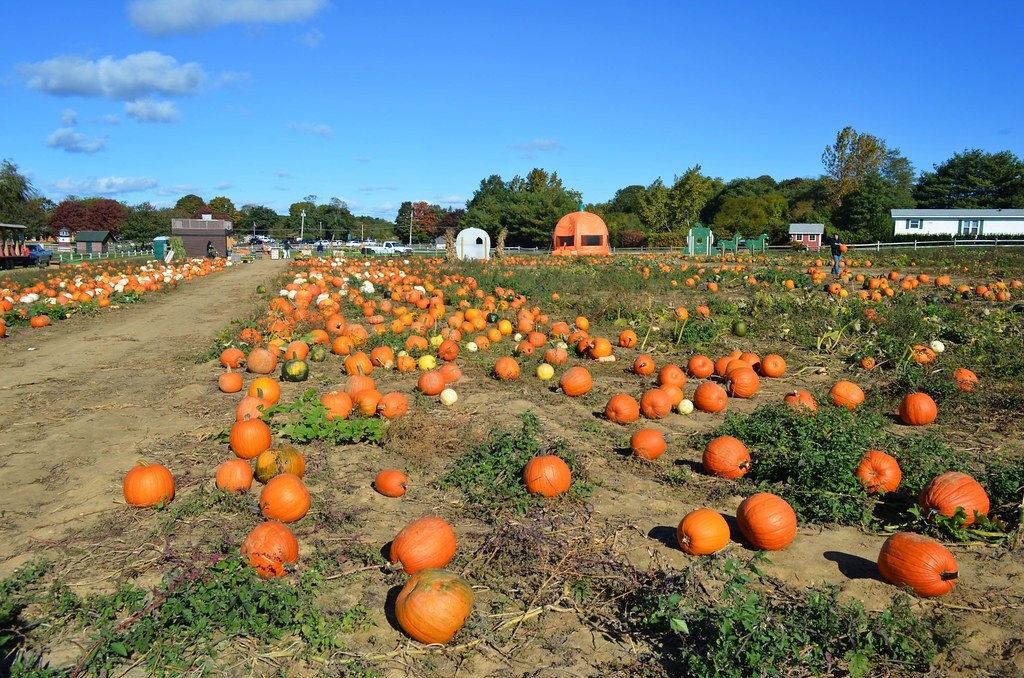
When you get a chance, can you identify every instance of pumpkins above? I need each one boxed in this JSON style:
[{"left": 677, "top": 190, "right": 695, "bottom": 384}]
[{"left": 0, "top": 244, "right": 1022, "bottom": 645}]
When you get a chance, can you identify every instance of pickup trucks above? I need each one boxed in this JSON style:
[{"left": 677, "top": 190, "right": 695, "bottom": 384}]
[
  {"left": 362, "top": 240, "right": 413, "bottom": 255},
  {"left": 24, "top": 243, "right": 53, "bottom": 267}
]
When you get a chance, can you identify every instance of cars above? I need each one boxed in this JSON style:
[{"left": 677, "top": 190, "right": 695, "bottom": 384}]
[
  {"left": 314, "top": 240, "right": 329, "bottom": 247},
  {"left": 302, "top": 238, "right": 314, "bottom": 244},
  {"left": 363, "top": 240, "right": 376, "bottom": 246},
  {"left": 346, "top": 241, "right": 361, "bottom": 247},
  {"left": 255, "top": 234, "right": 275, "bottom": 243},
  {"left": 332, "top": 240, "right": 342, "bottom": 247},
  {"left": 283, "top": 238, "right": 300, "bottom": 243},
  {"left": 353, "top": 239, "right": 362, "bottom": 243}
]
